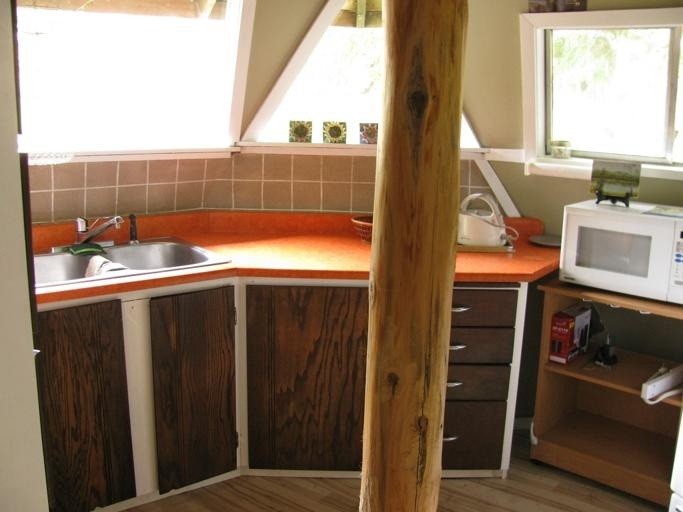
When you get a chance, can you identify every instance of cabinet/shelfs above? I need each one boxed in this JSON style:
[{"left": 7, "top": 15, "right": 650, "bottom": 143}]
[
  {"left": 34, "top": 299, "right": 136, "bottom": 512},
  {"left": 149, "top": 285, "right": 238, "bottom": 496},
  {"left": 530, "top": 280, "right": 682, "bottom": 507},
  {"left": 441, "top": 281, "right": 530, "bottom": 478},
  {"left": 246, "top": 285, "right": 368, "bottom": 471}
]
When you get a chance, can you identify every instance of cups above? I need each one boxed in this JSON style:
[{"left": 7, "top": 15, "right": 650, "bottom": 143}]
[
  {"left": 550, "top": 134, "right": 570, "bottom": 160},
  {"left": 526, "top": 0, "right": 555, "bottom": 12},
  {"left": 556, "top": 0, "right": 589, "bottom": 12}
]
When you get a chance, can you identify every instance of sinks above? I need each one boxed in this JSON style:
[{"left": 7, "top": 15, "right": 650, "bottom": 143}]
[
  {"left": 97, "top": 235, "right": 232, "bottom": 275},
  {"left": 32, "top": 248, "right": 124, "bottom": 288}
]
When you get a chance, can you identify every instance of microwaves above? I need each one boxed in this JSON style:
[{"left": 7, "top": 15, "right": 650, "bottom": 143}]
[{"left": 557, "top": 195, "right": 683, "bottom": 307}]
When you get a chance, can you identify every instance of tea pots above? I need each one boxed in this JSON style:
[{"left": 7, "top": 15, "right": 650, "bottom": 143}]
[{"left": 456, "top": 191, "right": 507, "bottom": 247}]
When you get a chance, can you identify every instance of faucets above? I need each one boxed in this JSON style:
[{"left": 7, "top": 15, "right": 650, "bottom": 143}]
[{"left": 48, "top": 216, "right": 125, "bottom": 254}]
